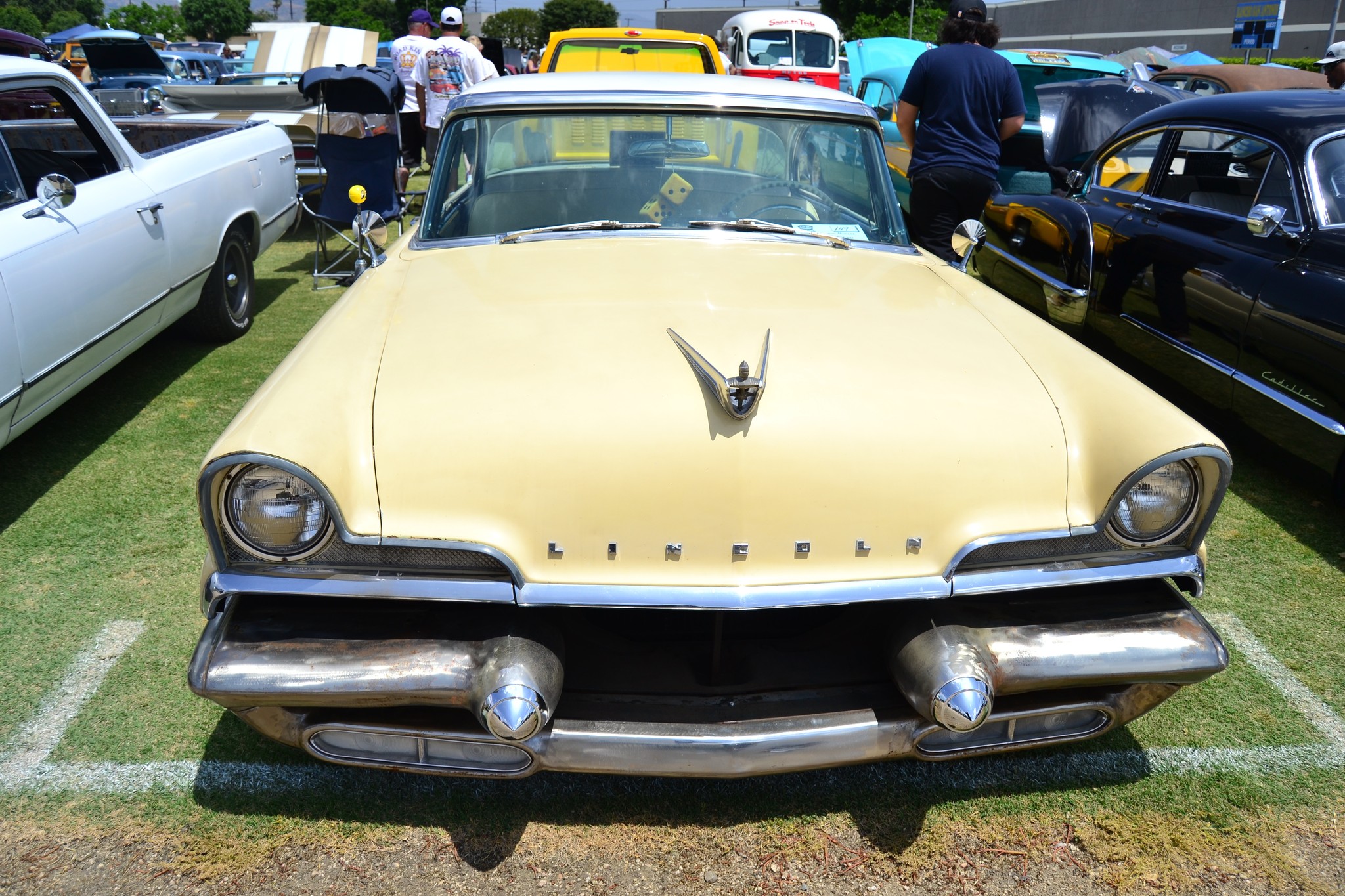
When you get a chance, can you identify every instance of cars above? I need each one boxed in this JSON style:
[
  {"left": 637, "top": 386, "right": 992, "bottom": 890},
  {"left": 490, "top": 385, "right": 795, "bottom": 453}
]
[
  {"left": 0, "top": 24, "right": 397, "bottom": 117},
  {"left": 1147, "top": 63, "right": 1333, "bottom": 96},
  {"left": 188, "top": 71, "right": 1234, "bottom": 782},
  {"left": 0, "top": 55, "right": 301, "bottom": 454},
  {"left": 794, "top": 37, "right": 1127, "bottom": 225},
  {"left": 955, "top": 76, "right": 1345, "bottom": 492},
  {"left": 514, "top": 27, "right": 785, "bottom": 190}
]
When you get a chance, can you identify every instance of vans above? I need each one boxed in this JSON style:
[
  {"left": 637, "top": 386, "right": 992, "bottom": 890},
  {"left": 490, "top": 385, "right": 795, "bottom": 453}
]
[{"left": 710, "top": 9, "right": 848, "bottom": 91}]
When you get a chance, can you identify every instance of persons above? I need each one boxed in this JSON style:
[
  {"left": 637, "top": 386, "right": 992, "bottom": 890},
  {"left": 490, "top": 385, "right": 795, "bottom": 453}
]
[
  {"left": 520, "top": 44, "right": 530, "bottom": 73},
  {"left": 461, "top": 35, "right": 501, "bottom": 183},
  {"left": 897, "top": 0, "right": 1027, "bottom": 259},
  {"left": 709, "top": 36, "right": 736, "bottom": 75},
  {"left": 524, "top": 52, "right": 540, "bottom": 73},
  {"left": 1312, "top": 41, "right": 1345, "bottom": 90},
  {"left": 410, "top": 5, "right": 493, "bottom": 196},
  {"left": 389, "top": 9, "right": 440, "bottom": 170}
]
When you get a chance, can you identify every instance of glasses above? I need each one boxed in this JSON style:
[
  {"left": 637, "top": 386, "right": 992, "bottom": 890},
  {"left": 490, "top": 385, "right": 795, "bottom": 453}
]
[{"left": 1323, "top": 61, "right": 1340, "bottom": 72}]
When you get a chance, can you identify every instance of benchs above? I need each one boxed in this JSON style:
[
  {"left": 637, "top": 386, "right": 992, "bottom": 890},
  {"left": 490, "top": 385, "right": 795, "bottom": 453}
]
[{"left": 469, "top": 188, "right": 804, "bottom": 233}]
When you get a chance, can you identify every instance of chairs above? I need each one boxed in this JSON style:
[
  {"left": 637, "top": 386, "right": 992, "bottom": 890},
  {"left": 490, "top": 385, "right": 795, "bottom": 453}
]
[
  {"left": 296, "top": 63, "right": 427, "bottom": 291},
  {"left": 1190, "top": 192, "right": 1297, "bottom": 221},
  {"left": 10, "top": 148, "right": 91, "bottom": 199}
]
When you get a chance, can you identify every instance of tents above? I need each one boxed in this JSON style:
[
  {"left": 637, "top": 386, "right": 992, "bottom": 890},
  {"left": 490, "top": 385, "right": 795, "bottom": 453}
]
[{"left": 1099, "top": 45, "right": 1301, "bottom": 86}]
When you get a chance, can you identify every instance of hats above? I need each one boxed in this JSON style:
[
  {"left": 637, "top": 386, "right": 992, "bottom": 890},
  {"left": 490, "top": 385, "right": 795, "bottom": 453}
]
[
  {"left": 408, "top": 9, "right": 440, "bottom": 28},
  {"left": 947, "top": 0, "right": 988, "bottom": 23},
  {"left": 440, "top": 6, "right": 463, "bottom": 25},
  {"left": 1312, "top": 40, "right": 1345, "bottom": 67}
]
